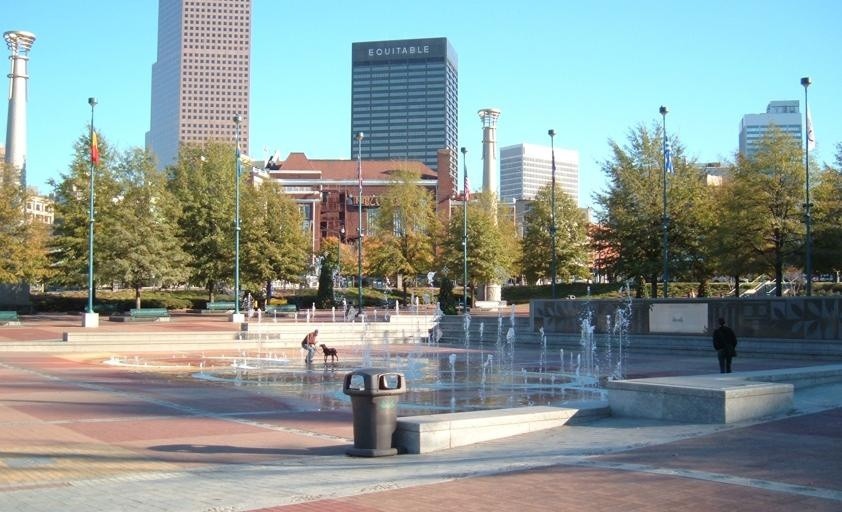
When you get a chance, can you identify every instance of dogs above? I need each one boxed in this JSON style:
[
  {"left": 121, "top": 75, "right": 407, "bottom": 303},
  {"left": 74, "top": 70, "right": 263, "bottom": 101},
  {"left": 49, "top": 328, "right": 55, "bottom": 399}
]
[{"left": 320, "top": 344, "right": 338, "bottom": 365}]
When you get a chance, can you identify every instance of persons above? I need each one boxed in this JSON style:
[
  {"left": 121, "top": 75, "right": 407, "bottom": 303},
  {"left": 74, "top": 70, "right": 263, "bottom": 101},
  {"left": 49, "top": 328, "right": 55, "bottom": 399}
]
[
  {"left": 712, "top": 316, "right": 738, "bottom": 374},
  {"left": 344, "top": 301, "right": 355, "bottom": 316},
  {"left": 242, "top": 290, "right": 254, "bottom": 311},
  {"left": 720, "top": 292, "right": 724, "bottom": 297},
  {"left": 689, "top": 287, "right": 696, "bottom": 299},
  {"left": 301, "top": 329, "right": 320, "bottom": 366}
]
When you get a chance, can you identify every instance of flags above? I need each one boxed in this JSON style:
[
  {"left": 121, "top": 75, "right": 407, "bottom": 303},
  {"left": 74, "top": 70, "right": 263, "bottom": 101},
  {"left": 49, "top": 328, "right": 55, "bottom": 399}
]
[
  {"left": 87, "top": 95, "right": 99, "bottom": 169},
  {"left": 660, "top": 103, "right": 677, "bottom": 177},
  {"left": 800, "top": 74, "right": 815, "bottom": 156},
  {"left": 234, "top": 112, "right": 242, "bottom": 182},
  {"left": 550, "top": 129, "right": 557, "bottom": 194},
  {"left": 461, "top": 145, "right": 469, "bottom": 204},
  {"left": 357, "top": 129, "right": 365, "bottom": 194}
]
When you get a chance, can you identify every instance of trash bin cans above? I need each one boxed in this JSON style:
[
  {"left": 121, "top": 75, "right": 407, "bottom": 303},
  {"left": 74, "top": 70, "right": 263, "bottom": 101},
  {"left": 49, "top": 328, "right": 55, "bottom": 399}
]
[{"left": 343, "top": 368, "right": 407, "bottom": 456}]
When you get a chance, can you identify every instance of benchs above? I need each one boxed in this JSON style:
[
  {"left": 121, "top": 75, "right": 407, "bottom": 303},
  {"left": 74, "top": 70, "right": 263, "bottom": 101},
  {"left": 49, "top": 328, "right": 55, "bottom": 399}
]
[
  {"left": 130, "top": 308, "right": 172, "bottom": 322},
  {"left": 0, "top": 310, "right": 19, "bottom": 328},
  {"left": 268, "top": 304, "right": 297, "bottom": 318},
  {"left": 207, "top": 302, "right": 236, "bottom": 313}
]
